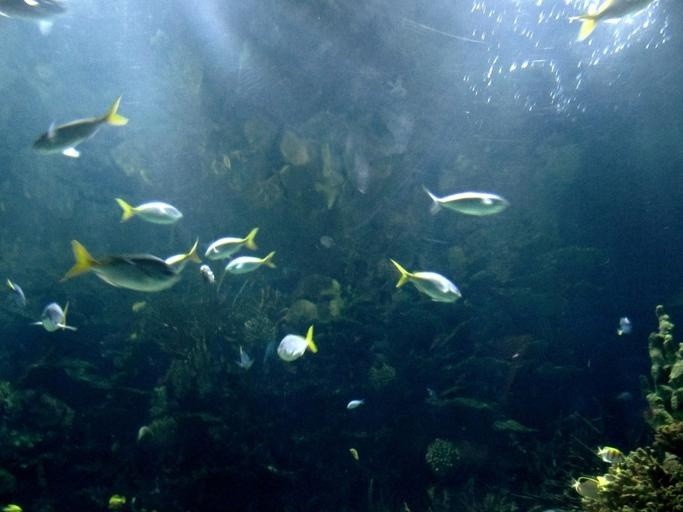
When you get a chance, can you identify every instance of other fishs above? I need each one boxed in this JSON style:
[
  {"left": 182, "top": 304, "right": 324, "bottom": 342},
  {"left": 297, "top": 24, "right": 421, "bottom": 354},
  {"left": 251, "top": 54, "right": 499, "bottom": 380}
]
[{"left": 0, "top": 96, "right": 630, "bottom": 512}]
